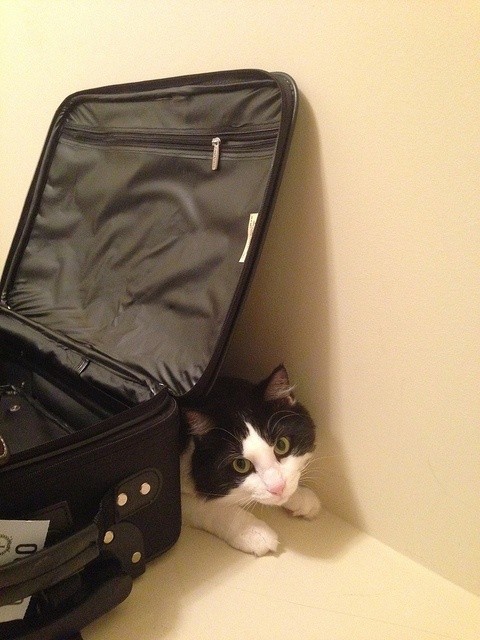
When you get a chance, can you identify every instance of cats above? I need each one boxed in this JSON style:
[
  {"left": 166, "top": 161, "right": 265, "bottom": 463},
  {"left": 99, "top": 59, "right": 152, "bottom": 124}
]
[{"left": 179, "top": 361, "right": 339, "bottom": 556}]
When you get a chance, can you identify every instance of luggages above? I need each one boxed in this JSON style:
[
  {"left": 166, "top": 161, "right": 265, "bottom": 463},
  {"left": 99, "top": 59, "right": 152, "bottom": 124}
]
[{"left": 1, "top": 67, "right": 297, "bottom": 633}]
